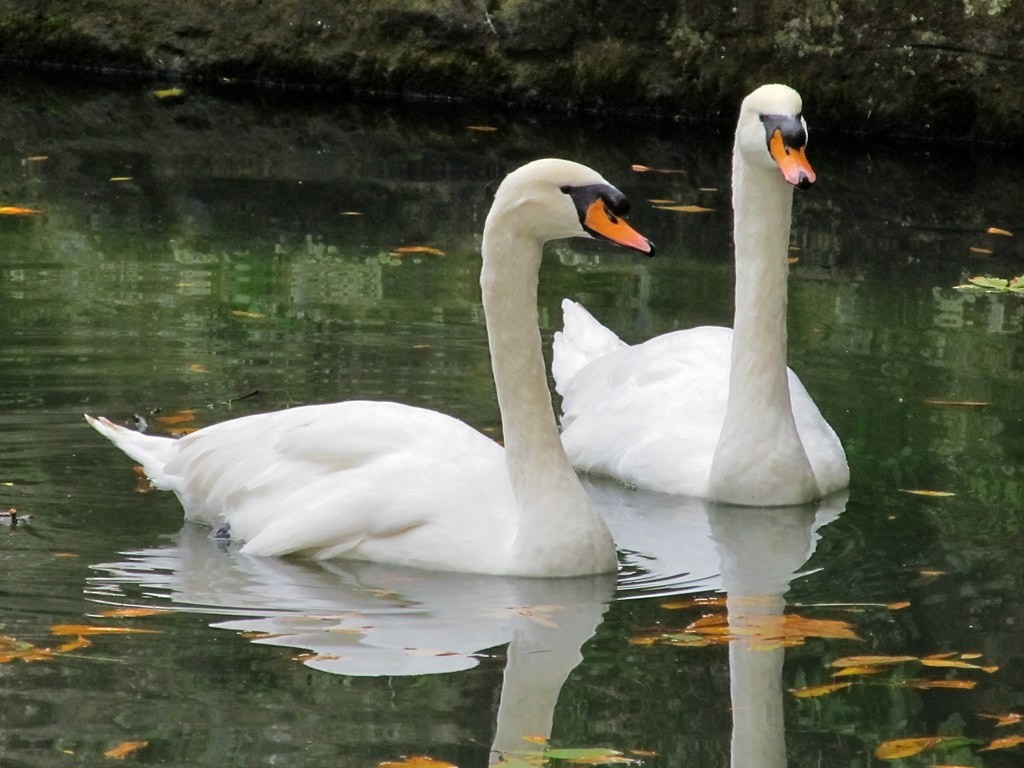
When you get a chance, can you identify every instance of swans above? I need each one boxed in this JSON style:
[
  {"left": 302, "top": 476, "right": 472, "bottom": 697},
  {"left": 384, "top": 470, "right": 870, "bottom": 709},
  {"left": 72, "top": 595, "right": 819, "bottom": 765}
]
[
  {"left": 553, "top": 83, "right": 850, "bottom": 505},
  {"left": 84, "top": 158, "right": 655, "bottom": 575}
]
